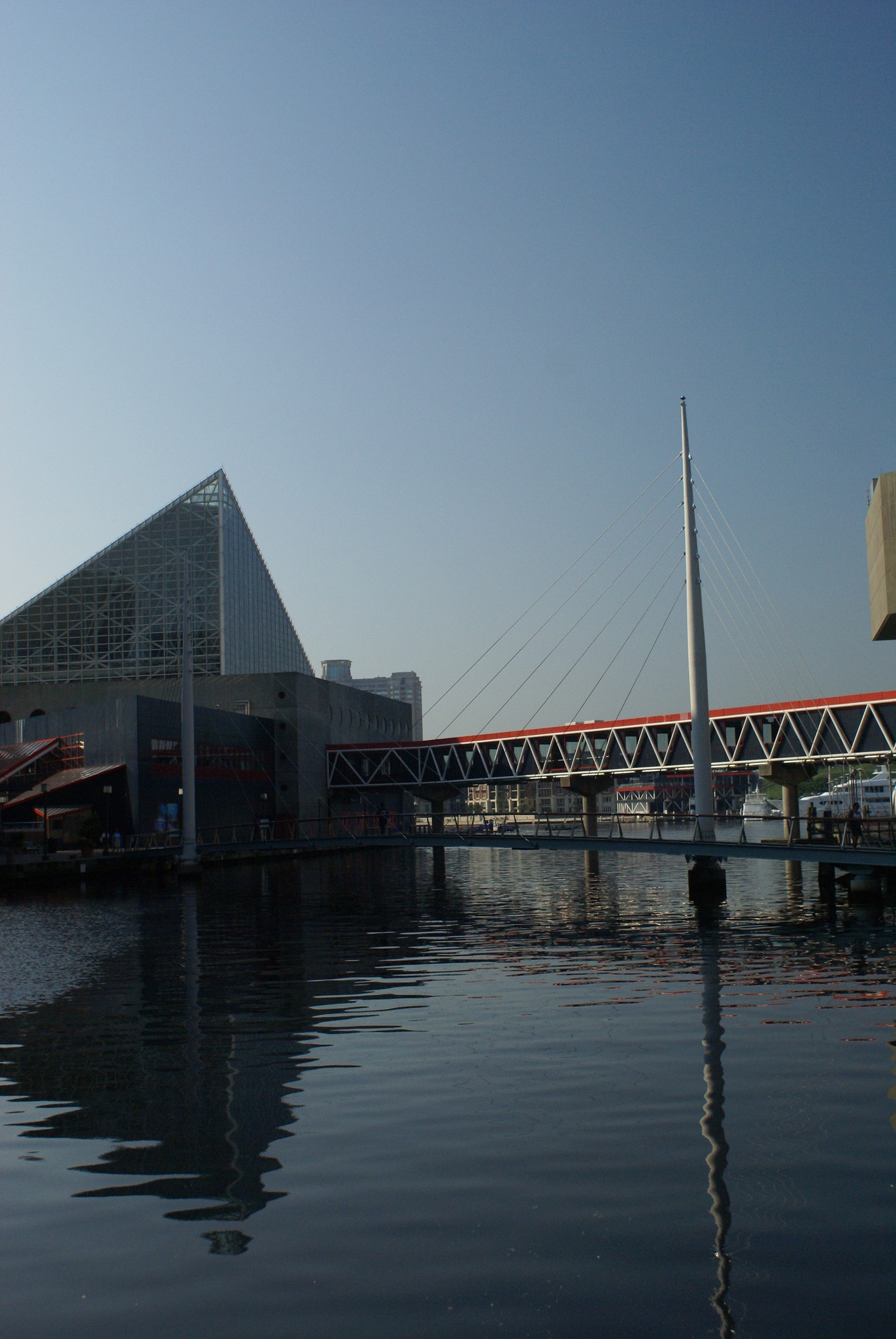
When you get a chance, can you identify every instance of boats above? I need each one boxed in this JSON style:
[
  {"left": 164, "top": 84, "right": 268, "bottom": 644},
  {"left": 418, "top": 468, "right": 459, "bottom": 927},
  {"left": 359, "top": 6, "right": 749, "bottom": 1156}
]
[
  {"left": 459, "top": 817, "right": 520, "bottom": 834},
  {"left": 743, "top": 784, "right": 785, "bottom": 821},
  {"left": 800, "top": 763, "right": 896, "bottom": 820},
  {"left": 544, "top": 824, "right": 578, "bottom": 831}
]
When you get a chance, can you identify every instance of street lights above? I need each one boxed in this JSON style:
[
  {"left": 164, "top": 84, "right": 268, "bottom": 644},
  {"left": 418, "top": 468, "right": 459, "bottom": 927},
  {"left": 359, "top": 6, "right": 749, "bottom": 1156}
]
[
  {"left": 844, "top": 773, "right": 851, "bottom": 809},
  {"left": 830, "top": 781, "right": 835, "bottom": 814},
  {"left": 833, "top": 778, "right": 840, "bottom": 814},
  {"left": 260, "top": 792, "right": 267, "bottom": 840},
  {"left": 456, "top": 799, "right": 459, "bottom": 826},
  {"left": 40, "top": 783, "right": 50, "bottom": 862},
  {"left": 465, "top": 799, "right": 469, "bottom": 828},
  {"left": 856, "top": 768, "right": 863, "bottom": 818},
  {"left": 839, "top": 776, "right": 845, "bottom": 813},
  {"left": 103, "top": 785, "right": 110, "bottom": 856},
  {"left": 414, "top": 802, "right": 418, "bottom": 825}
]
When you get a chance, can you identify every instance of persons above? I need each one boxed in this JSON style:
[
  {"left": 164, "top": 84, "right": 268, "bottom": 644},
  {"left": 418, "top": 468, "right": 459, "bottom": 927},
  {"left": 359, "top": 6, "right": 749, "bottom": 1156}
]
[
  {"left": 377, "top": 803, "right": 388, "bottom": 833},
  {"left": 848, "top": 802, "right": 862, "bottom": 847},
  {"left": 101, "top": 828, "right": 121, "bottom": 848}
]
